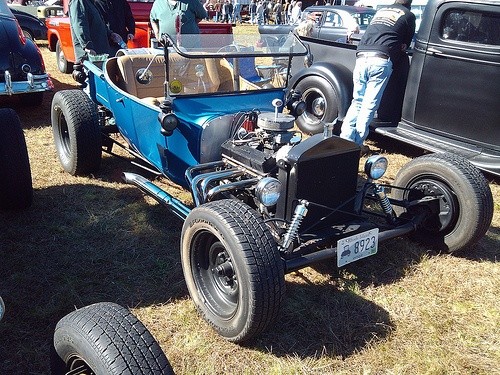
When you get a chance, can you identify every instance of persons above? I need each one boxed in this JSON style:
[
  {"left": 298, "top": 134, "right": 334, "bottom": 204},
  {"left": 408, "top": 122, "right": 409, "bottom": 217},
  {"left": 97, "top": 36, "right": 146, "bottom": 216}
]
[
  {"left": 150, "top": 0, "right": 206, "bottom": 48},
  {"left": 340, "top": 0, "right": 416, "bottom": 156},
  {"left": 202, "top": 0, "right": 331, "bottom": 25},
  {"left": 92, "top": 0, "right": 135, "bottom": 52},
  {"left": 68, "top": 0, "right": 122, "bottom": 97}
]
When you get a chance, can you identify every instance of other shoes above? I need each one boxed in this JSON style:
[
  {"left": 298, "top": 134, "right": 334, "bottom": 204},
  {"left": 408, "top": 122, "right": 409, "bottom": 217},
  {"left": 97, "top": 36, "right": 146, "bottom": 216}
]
[{"left": 361, "top": 146, "right": 369, "bottom": 156}]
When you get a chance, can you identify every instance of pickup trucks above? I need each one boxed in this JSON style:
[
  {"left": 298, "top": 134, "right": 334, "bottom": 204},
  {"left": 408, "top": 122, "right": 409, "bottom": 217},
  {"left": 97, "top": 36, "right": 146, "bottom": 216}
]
[
  {"left": 257, "top": 0, "right": 500, "bottom": 178},
  {"left": 45, "top": 0, "right": 236, "bottom": 74}
]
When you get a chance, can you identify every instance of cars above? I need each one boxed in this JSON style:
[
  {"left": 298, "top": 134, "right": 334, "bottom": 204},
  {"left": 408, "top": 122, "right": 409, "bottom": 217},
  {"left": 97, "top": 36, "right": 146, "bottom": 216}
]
[
  {"left": 0, "top": 0, "right": 53, "bottom": 94},
  {"left": 50, "top": 30, "right": 494, "bottom": 343}
]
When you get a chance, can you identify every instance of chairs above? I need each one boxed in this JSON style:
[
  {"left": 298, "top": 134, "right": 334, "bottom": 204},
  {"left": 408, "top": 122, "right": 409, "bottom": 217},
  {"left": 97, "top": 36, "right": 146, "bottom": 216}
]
[{"left": 229, "top": 45, "right": 282, "bottom": 85}]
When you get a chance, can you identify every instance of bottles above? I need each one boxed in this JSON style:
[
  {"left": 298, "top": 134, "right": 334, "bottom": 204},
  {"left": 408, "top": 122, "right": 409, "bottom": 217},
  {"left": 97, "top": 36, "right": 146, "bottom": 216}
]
[{"left": 118, "top": 40, "right": 128, "bottom": 49}]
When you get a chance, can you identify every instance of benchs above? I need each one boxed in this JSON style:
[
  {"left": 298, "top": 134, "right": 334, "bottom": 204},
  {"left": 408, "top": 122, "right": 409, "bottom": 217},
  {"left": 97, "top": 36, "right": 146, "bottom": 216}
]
[{"left": 117, "top": 52, "right": 234, "bottom": 105}]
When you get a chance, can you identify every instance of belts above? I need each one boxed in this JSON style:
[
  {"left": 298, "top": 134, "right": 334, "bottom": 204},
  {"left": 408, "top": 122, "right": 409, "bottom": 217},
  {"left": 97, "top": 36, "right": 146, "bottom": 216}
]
[{"left": 356, "top": 53, "right": 389, "bottom": 59}]
